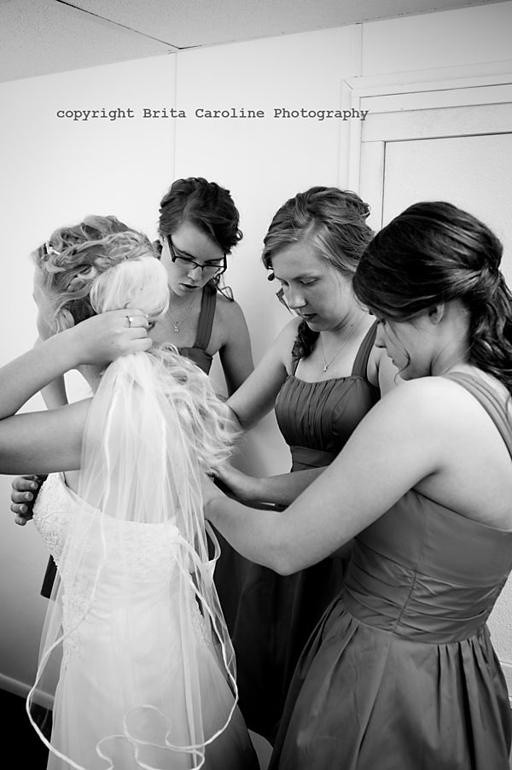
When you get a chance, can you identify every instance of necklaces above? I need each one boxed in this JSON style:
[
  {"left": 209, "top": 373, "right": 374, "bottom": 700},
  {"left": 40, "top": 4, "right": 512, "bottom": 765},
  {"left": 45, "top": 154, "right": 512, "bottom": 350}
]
[
  {"left": 161, "top": 296, "right": 196, "bottom": 333},
  {"left": 318, "top": 324, "right": 355, "bottom": 374}
]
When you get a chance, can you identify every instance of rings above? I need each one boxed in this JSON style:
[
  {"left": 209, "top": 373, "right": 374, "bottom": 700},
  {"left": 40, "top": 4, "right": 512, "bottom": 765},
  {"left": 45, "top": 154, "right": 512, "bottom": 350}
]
[{"left": 127, "top": 313, "right": 134, "bottom": 330}]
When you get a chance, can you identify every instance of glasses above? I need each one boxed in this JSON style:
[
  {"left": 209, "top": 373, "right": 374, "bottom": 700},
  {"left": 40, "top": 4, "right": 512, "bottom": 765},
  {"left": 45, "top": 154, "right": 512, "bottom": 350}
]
[{"left": 165, "top": 231, "right": 229, "bottom": 277}]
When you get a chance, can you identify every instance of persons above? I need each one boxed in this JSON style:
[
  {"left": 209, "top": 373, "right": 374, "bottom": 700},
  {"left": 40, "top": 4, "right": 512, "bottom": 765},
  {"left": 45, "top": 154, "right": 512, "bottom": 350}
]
[
  {"left": 188, "top": 184, "right": 412, "bottom": 770},
  {"left": 195, "top": 202, "right": 511, "bottom": 766},
  {"left": 1, "top": 216, "right": 263, "bottom": 769},
  {"left": 34, "top": 176, "right": 257, "bottom": 410}
]
